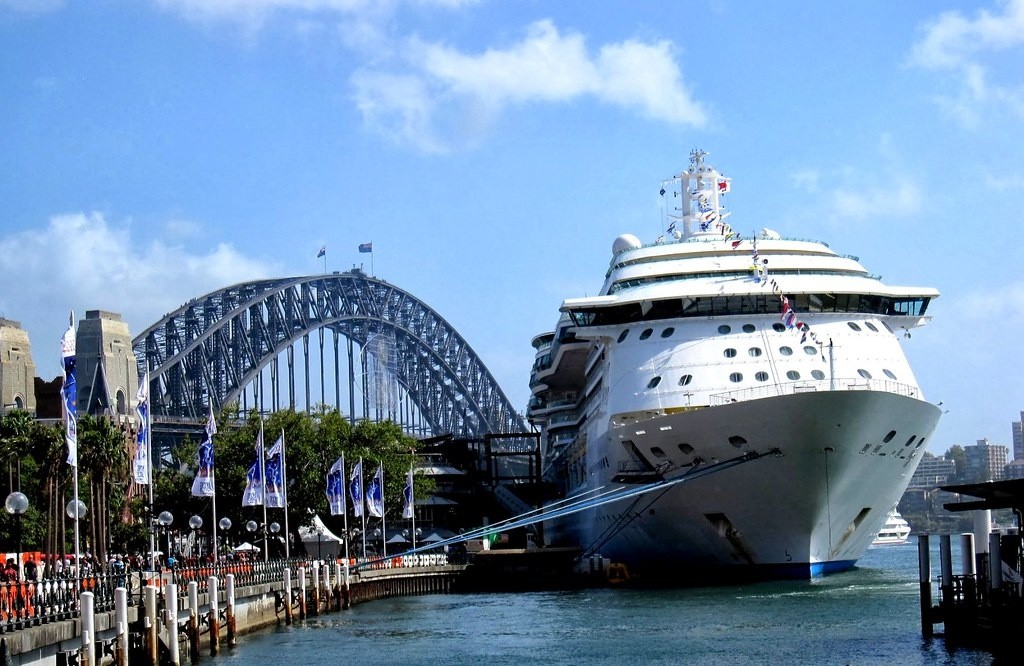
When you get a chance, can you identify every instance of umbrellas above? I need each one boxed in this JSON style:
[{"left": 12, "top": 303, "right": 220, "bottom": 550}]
[{"left": 386, "top": 532, "right": 445, "bottom": 554}]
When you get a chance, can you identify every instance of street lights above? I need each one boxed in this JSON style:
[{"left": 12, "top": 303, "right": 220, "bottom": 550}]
[
  {"left": 246, "top": 520, "right": 258, "bottom": 564},
  {"left": 315, "top": 525, "right": 324, "bottom": 558},
  {"left": 269, "top": 522, "right": 281, "bottom": 563},
  {"left": 373, "top": 528, "right": 382, "bottom": 557},
  {"left": 415, "top": 527, "right": 422, "bottom": 548},
  {"left": 5, "top": 491, "right": 29, "bottom": 583},
  {"left": 353, "top": 527, "right": 360, "bottom": 564},
  {"left": 401, "top": 529, "right": 409, "bottom": 553},
  {"left": 189, "top": 514, "right": 202, "bottom": 568},
  {"left": 219, "top": 517, "right": 232, "bottom": 565},
  {"left": 66, "top": 498, "right": 87, "bottom": 576},
  {"left": 158, "top": 511, "right": 174, "bottom": 568}
]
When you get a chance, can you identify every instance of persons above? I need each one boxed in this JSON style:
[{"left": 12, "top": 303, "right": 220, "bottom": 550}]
[
  {"left": 166, "top": 548, "right": 259, "bottom": 569},
  {"left": 23, "top": 556, "right": 37, "bottom": 582},
  {"left": 56, "top": 556, "right": 70, "bottom": 576},
  {"left": 0, "top": 558, "right": 20, "bottom": 582},
  {"left": 81, "top": 552, "right": 161, "bottom": 578}
]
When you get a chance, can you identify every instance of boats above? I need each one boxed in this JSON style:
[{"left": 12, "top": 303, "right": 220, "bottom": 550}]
[
  {"left": 870, "top": 508, "right": 912, "bottom": 547},
  {"left": 523, "top": 144, "right": 946, "bottom": 583}
]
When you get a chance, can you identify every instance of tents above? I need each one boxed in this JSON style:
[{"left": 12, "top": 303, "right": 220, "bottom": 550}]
[
  {"left": 232, "top": 542, "right": 261, "bottom": 553},
  {"left": 273, "top": 514, "right": 343, "bottom": 558}
]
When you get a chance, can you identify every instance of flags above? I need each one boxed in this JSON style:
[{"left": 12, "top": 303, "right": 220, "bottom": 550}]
[
  {"left": 367, "top": 467, "right": 383, "bottom": 517},
  {"left": 318, "top": 248, "right": 325, "bottom": 257},
  {"left": 403, "top": 472, "right": 412, "bottom": 518},
  {"left": 326, "top": 460, "right": 344, "bottom": 516},
  {"left": 359, "top": 243, "right": 372, "bottom": 253},
  {"left": 265, "top": 439, "right": 285, "bottom": 507},
  {"left": 135, "top": 374, "right": 149, "bottom": 484},
  {"left": 242, "top": 430, "right": 264, "bottom": 506},
  {"left": 191, "top": 414, "right": 218, "bottom": 497},
  {"left": 61, "top": 327, "right": 77, "bottom": 466},
  {"left": 350, "top": 463, "right": 363, "bottom": 517}
]
